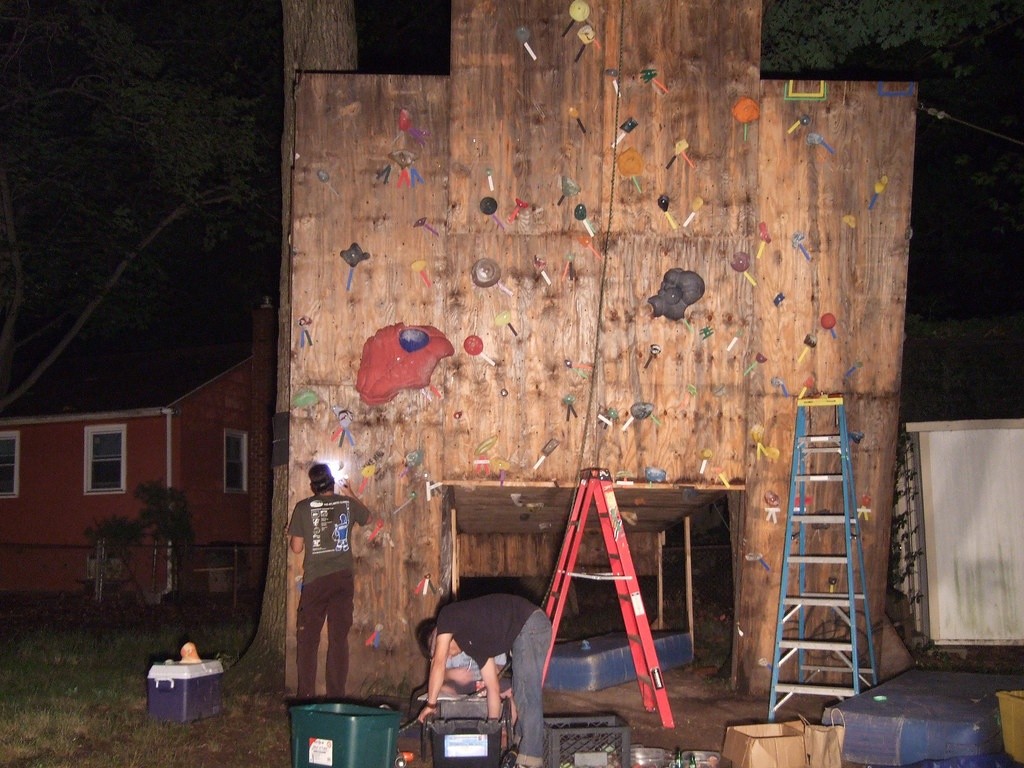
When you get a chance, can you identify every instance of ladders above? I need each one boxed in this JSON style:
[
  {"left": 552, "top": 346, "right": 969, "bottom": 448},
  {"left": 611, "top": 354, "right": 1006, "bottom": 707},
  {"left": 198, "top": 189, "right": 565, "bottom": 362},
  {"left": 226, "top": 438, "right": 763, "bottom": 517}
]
[
  {"left": 767, "top": 397, "right": 877, "bottom": 721},
  {"left": 533, "top": 469, "right": 674, "bottom": 729}
]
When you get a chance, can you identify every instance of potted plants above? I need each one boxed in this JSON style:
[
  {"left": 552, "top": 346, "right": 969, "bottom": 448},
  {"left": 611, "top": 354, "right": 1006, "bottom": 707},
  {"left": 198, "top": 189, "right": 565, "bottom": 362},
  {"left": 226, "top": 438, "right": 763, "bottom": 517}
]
[
  {"left": 86, "top": 516, "right": 145, "bottom": 580},
  {"left": 193, "top": 541, "right": 248, "bottom": 597}
]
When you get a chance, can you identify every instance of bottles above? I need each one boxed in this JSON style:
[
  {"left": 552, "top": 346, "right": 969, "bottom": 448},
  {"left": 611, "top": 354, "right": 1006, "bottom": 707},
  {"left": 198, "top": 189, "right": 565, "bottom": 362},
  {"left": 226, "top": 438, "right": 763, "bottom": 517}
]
[
  {"left": 395, "top": 753, "right": 407, "bottom": 768},
  {"left": 675, "top": 751, "right": 685, "bottom": 768},
  {"left": 689, "top": 754, "right": 696, "bottom": 768}
]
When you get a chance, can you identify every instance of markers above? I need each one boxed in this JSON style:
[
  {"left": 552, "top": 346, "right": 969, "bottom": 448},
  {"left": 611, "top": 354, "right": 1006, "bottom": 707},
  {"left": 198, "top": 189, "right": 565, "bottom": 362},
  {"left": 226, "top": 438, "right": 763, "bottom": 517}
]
[{"left": 340, "top": 480, "right": 347, "bottom": 487}]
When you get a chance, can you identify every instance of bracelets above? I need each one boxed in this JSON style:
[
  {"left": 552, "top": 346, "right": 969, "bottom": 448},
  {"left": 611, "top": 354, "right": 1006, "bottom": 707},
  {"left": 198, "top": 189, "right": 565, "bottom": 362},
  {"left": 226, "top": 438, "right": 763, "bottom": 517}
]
[
  {"left": 425, "top": 702, "right": 437, "bottom": 709},
  {"left": 488, "top": 717, "right": 499, "bottom": 720}
]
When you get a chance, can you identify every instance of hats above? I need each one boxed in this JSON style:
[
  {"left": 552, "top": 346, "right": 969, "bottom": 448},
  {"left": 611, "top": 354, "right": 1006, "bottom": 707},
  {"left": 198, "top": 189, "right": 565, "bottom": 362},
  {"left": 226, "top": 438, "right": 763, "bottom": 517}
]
[{"left": 308, "top": 464, "right": 332, "bottom": 483}]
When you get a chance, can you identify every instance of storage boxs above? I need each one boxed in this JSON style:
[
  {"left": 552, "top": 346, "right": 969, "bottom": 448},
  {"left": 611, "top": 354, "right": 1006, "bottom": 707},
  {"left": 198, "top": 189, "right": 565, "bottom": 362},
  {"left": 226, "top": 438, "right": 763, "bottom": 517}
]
[
  {"left": 289, "top": 702, "right": 403, "bottom": 768},
  {"left": 541, "top": 714, "right": 630, "bottom": 768},
  {"left": 630, "top": 744, "right": 721, "bottom": 768},
  {"left": 721, "top": 721, "right": 809, "bottom": 768},
  {"left": 995, "top": 690, "right": 1024, "bottom": 762},
  {"left": 151, "top": 600, "right": 184, "bottom": 626},
  {"left": 406, "top": 669, "right": 516, "bottom": 768},
  {"left": 147, "top": 658, "right": 224, "bottom": 723}
]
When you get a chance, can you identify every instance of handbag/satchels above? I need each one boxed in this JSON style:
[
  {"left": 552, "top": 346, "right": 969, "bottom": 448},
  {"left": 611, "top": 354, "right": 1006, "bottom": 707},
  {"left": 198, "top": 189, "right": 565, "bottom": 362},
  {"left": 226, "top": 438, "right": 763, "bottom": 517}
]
[{"left": 798, "top": 708, "right": 845, "bottom": 768}]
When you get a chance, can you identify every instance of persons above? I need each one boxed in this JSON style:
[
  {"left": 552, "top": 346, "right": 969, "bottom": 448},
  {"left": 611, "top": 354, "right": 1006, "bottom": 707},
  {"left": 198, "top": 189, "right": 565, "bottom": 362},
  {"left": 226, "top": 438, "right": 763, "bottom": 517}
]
[
  {"left": 418, "top": 592, "right": 551, "bottom": 768},
  {"left": 287, "top": 465, "right": 372, "bottom": 703}
]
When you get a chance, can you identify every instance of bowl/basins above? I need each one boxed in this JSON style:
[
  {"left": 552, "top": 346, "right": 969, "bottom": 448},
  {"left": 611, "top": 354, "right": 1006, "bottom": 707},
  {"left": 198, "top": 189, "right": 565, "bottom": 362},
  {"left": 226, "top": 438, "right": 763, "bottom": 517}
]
[
  {"left": 630, "top": 748, "right": 665, "bottom": 768},
  {"left": 681, "top": 751, "right": 721, "bottom": 768}
]
